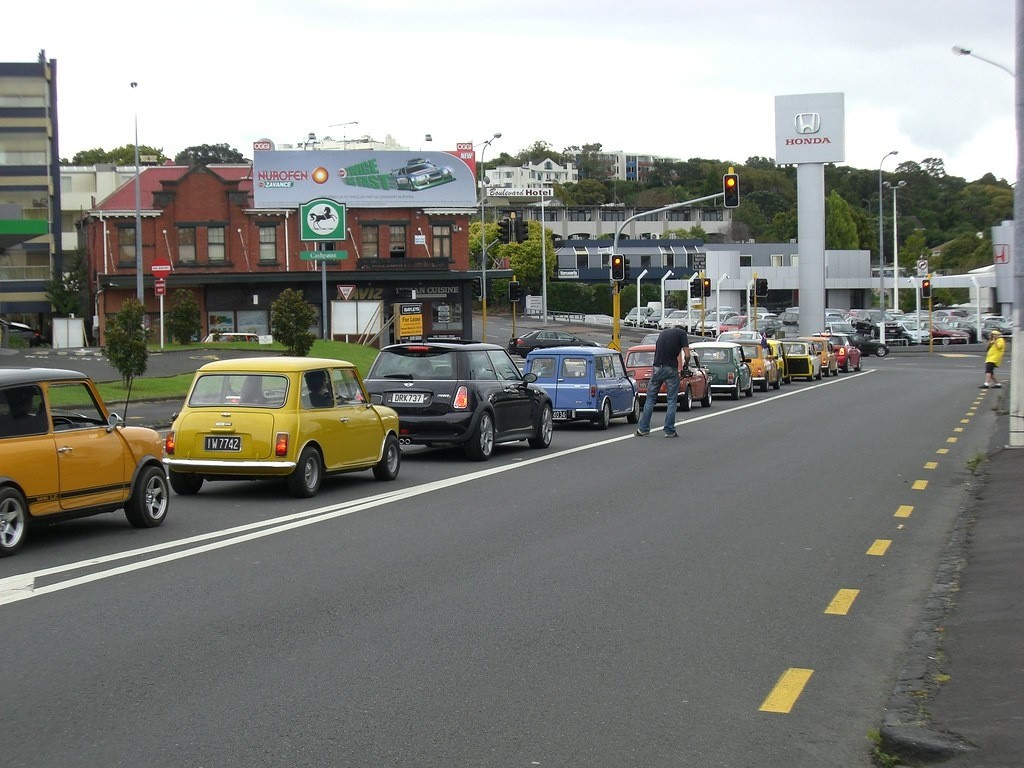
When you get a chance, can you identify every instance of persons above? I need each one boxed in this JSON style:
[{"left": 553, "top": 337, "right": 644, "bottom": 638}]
[
  {"left": 978, "top": 330, "right": 1007, "bottom": 388},
  {"left": 303, "top": 372, "right": 334, "bottom": 407},
  {"left": 238, "top": 375, "right": 268, "bottom": 405},
  {"left": 635, "top": 323, "right": 691, "bottom": 437}
]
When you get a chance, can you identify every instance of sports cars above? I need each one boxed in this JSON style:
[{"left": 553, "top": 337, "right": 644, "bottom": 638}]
[{"left": 395, "top": 163, "right": 456, "bottom": 191}]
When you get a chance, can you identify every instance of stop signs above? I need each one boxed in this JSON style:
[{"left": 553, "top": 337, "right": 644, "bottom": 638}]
[{"left": 150, "top": 257, "right": 171, "bottom": 278}]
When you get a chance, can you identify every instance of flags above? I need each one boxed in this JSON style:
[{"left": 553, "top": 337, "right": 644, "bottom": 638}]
[{"left": 760, "top": 332, "right": 768, "bottom": 350}]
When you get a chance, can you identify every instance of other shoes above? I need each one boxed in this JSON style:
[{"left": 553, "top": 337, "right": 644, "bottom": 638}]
[
  {"left": 664, "top": 430, "right": 678, "bottom": 437},
  {"left": 990, "top": 384, "right": 1002, "bottom": 389},
  {"left": 636, "top": 428, "right": 650, "bottom": 436},
  {"left": 979, "top": 384, "right": 990, "bottom": 389}
]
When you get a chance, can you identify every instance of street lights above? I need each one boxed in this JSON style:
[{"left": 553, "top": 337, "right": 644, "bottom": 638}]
[
  {"left": 482, "top": 131, "right": 503, "bottom": 342},
  {"left": 878, "top": 151, "right": 899, "bottom": 343},
  {"left": 523, "top": 164, "right": 549, "bottom": 326},
  {"left": 883, "top": 180, "right": 908, "bottom": 314},
  {"left": 130, "top": 81, "right": 146, "bottom": 343}
]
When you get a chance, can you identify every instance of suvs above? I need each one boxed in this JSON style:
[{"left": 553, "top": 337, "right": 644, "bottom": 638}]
[{"left": 354, "top": 339, "right": 554, "bottom": 461}]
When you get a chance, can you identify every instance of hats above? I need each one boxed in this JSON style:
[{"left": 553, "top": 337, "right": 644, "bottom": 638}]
[{"left": 991, "top": 330, "right": 1000, "bottom": 334}]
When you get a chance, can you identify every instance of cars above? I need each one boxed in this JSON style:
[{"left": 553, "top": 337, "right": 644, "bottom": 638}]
[
  {"left": 507, "top": 329, "right": 602, "bottom": 360},
  {"left": 200, "top": 332, "right": 259, "bottom": 344},
  {"left": 0, "top": 315, "right": 41, "bottom": 348},
  {"left": 407, "top": 158, "right": 436, "bottom": 167},
  {"left": 0, "top": 366, "right": 171, "bottom": 559},
  {"left": 623, "top": 306, "right": 1013, "bottom": 401},
  {"left": 623, "top": 343, "right": 712, "bottom": 412},
  {"left": 521, "top": 346, "right": 641, "bottom": 430},
  {"left": 162, "top": 356, "right": 405, "bottom": 498}
]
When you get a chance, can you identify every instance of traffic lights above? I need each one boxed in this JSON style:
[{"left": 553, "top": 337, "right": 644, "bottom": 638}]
[
  {"left": 471, "top": 276, "right": 483, "bottom": 298},
  {"left": 509, "top": 281, "right": 523, "bottom": 301},
  {"left": 497, "top": 217, "right": 511, "bottom": 244},
  {"left": 690, "top": 278, "right": 701, "bottom": 298},
  {"left": 610, "top": 253, "right": 625, "bottom": 282},
  {"left": 723, "top": 174, "right": 740, "bottom": 209},
  {"left": 922, "top": 280, "right": 932, "bottom": 298},
  {"left": 515, "top": 217, "right": 529, "bottom": 244},
  {"left": 703, "top": 278, "right": 711, "bottom": 298},
  {"left": 754, "top": 278, "right": 769, "bottom": 298},
  {"left": 620, "top": 254, "right": 631, "bottom": 289}
]
